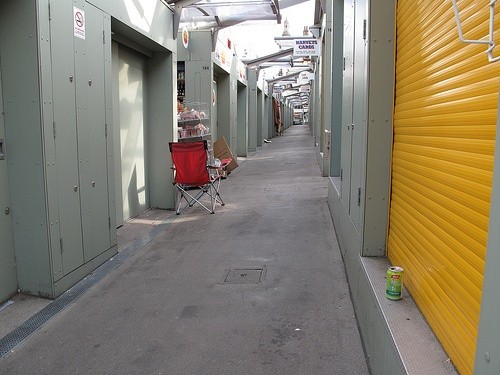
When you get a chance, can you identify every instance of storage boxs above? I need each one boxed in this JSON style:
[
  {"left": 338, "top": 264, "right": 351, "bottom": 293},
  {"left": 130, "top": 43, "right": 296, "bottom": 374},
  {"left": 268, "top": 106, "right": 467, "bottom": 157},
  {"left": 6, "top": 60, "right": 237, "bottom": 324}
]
[{"left": 213, "top": 136, "right": 238, "bottom": 174}]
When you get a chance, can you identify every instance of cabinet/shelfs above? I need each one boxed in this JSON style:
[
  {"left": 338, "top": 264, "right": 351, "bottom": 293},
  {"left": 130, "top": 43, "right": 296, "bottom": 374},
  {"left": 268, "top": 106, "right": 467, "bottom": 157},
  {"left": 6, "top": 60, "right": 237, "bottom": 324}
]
[
  {"left": 177, "top": 69, "right": 185, "bottom": 108},
  {"left": 176, "top": 117, "right": 213, "bottom": 176}
]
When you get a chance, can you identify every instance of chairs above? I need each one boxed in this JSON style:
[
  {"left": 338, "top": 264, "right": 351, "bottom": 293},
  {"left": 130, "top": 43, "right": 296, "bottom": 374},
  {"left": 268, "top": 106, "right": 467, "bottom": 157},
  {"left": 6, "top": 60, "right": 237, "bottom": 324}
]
[{"left": 169, "top": 139, "right": 225, "bottom": 215}]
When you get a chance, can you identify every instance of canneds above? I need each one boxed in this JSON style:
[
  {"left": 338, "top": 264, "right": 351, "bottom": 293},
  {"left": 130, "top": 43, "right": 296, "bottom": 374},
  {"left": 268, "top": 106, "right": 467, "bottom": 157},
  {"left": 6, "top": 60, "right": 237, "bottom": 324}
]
[{"left": 384, "top": 266, "right": 405, "bottom": 301}]
